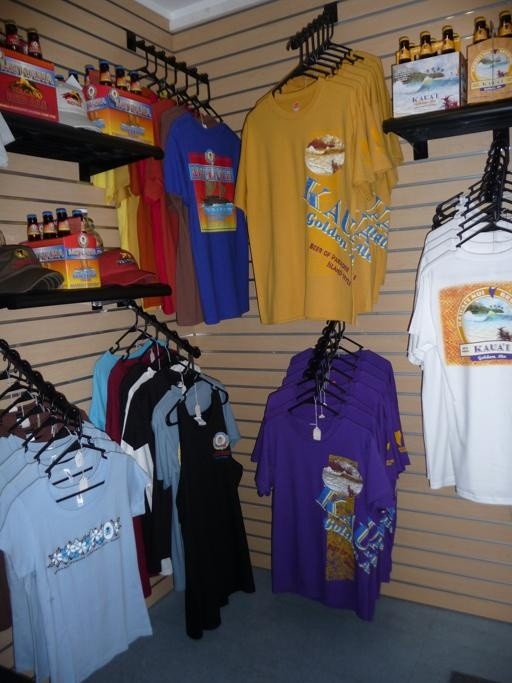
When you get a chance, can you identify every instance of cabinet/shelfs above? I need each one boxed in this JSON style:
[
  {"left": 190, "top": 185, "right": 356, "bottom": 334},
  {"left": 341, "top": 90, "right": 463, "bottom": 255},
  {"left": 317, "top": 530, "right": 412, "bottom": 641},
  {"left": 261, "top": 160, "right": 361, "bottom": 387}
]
[{"left": 1, "top": 109, "right": 172, "bottom": 309}]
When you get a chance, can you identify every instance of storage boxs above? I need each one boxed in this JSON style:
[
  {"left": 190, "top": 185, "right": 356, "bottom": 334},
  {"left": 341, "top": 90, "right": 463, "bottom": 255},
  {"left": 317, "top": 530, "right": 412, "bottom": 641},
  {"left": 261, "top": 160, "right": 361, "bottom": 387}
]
[
  {"left": 18, "top": 232, "right": 102, "bottom": 290},
  {"left": 0, "top": 46, "right": 63, "bottom": 122},
  {"left": 465, "top": 36, "right": 512, "bottom": 105},
  {"left": 81, "top": 70, "right": 155, "bottom": 145},
  {"left": 391, "top": 50, "right": 466, "bottom": 118}
]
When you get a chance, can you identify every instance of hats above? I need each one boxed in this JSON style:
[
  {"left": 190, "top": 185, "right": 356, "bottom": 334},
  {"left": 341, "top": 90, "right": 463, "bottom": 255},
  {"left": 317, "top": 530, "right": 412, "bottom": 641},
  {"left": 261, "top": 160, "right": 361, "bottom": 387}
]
[
  {"left": 1, "top": 244, "right": 64, "bottom": 294},
  {"left": 96, "top": 247, "right": 160, "bottom": 288}
]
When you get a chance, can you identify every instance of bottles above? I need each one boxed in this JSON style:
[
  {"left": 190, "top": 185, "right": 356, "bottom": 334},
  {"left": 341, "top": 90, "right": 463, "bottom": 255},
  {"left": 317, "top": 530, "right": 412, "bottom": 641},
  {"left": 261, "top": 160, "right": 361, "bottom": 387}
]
[
  {"left": 129, "top": 71, "right": 143, "bottom": 96},
  {"left": 420, "top": 31, "right": 433, "bottom": 59},
  {"left": 84, "top": 65, "right": 97, "bottom": 84},
  {"left": 474, "top": 16, "right": 489, "bottom": 44},
  {"left": 2, "top": 20, "right": 25, "bottom": 54},
  {"left": 24, "top": 28, "right": 42, "bottom": 59},
  {"left": 43, "top": 211, "right": 58, "bottom": 238},
  {"left": 498, "top": 11, "right": 512, "bottom": 37},
  {"left": 73, "top": 208, "right": 86, "bottom": 234},
  {"left": 115, "top": 66, "right": 127, "bottom": 90},
  {"left": 99, "top": 61, "right": 112, "bottom": 86},
  {"left": 441, "top": 25, "right": 455, "bottom": 54},
  {"left": 399, "top": 36, "right": 411, "bottom": 64},
  {"left": 57, "top": 208, "right": 72, "bottom": 237},
  {"left": 27, "top": 215, "right": 41, "bottom": 241},
  {"left": 67, "top": 70, "right": 79, "bottom": 85}
]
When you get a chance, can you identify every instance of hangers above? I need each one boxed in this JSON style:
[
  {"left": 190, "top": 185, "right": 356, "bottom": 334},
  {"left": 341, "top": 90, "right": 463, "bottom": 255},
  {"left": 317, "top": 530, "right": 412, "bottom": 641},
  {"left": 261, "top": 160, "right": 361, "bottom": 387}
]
[
  {"left": 109, "top": 304, "right": 232, "bottom": 428},
  {"left": 271, "top": 11, "right": 355, "bottom": 99},
  {"left": 124, "top": 39, "right": 226, "bottom": 127},
  {"left": 432, "top": 128, "right": 512, "bottom": 259},
  {"left": 0, "top": 337, "right": 111, "bottom": 506},
  {"left": 287, "top": 316, "right": 364, "bottom": 419}
]
